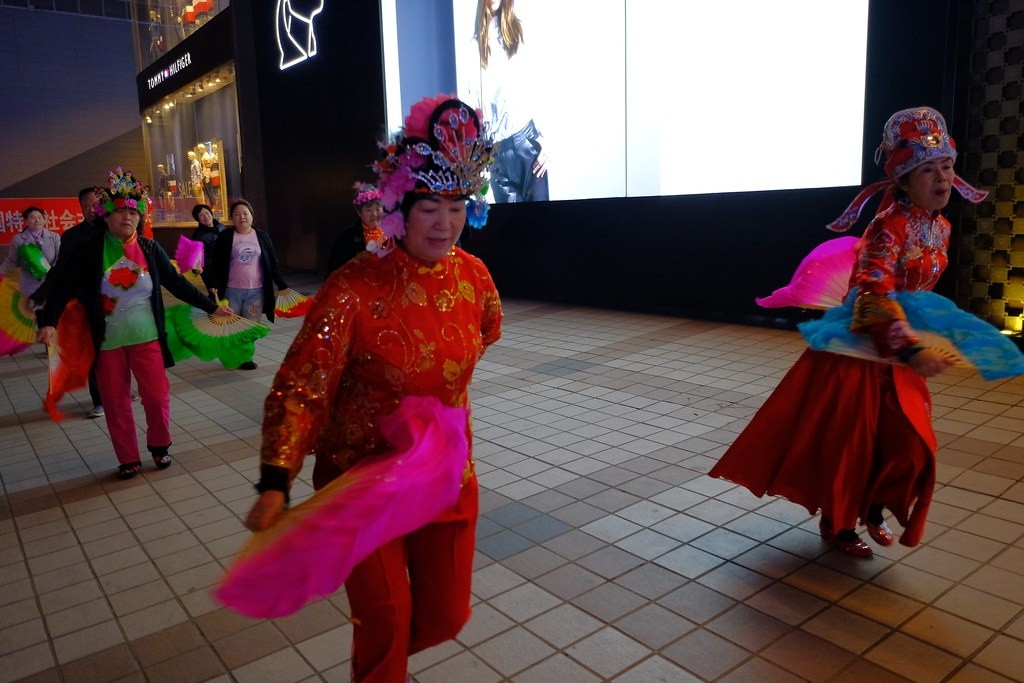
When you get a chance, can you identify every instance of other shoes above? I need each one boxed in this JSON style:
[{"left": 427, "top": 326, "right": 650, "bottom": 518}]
[
  {"left": 817, "top": 523, "right": 871, "bottom": 556},
  {"left": 152, "top": 450, "right": 171, "bottom": 468},
  {"left": 88, "top": 406, "right": 104, "bottom": 418},
  {"left": 241, "top": 361, "right": 257, "bottom": 369},
  {"left": 119, "top": 465, "right": 140, "bottom": 478},
  {"left": 868, "top": 521, "right": 893, "bottom": 547}
]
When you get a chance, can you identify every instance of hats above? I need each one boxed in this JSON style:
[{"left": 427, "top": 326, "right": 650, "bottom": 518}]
[{"left": 826, "top": 107, "right": 990, "bottom": 233}]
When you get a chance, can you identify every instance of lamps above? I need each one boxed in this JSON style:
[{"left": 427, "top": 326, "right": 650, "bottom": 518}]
[{"left": 186, "top": 71, "right": 220, "bottom": 97}]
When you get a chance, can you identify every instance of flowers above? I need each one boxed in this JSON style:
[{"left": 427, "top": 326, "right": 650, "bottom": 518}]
[
  {"left": 354, "top": 190, "right": 382, "bottom": 205},
  {"left": 376, "top": 93, "right": 492, "bottom": 239},
  {"left": 95, "top": 198, "right": 148, "bottom": 214}
]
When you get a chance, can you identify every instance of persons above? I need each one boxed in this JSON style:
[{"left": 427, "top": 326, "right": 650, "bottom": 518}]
[
  {"left": 209, "top": 199, "right": 290, "bottom": 370},
  {"left": 351, "top": 181, "right": 386, "bottom": 256},
  {"left": 466, "top": 0, "right": 550, "bottom": 204},
  {"left": 707, "top": 106, "right": 990, "bottom": 555},
  {"left": 191, "top": 204, "right": 227, "bottom": 291},
  {"left": 29, "top": 187, "right": 137, "bottom": 418},
  {"left": 0, "top": 207, "right": 62, "bottom": 295},
  {"left": 148, "top": 10, "right": 163, "bottom": 62},
  {"left": 209, "top": 94, "right": 504, "bottom": 683},
  {"left": 143, "top": 142, "right": 221, "bottom": 212}
]
